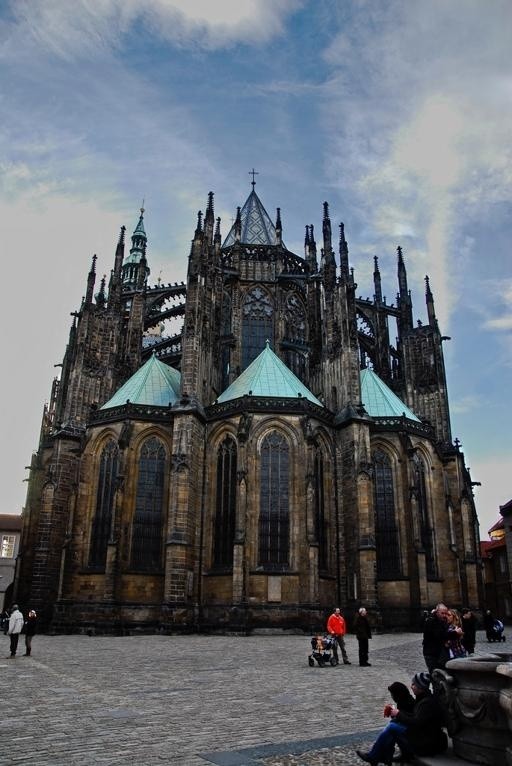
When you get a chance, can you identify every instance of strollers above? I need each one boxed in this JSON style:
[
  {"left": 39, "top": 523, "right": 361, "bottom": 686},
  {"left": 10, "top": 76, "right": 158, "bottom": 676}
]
[
  {"left": 308, "top": 633, "right": 339, "bottom": 666},
  {"left": 491, "top": 619, "right": 506, "bottom": 642}
]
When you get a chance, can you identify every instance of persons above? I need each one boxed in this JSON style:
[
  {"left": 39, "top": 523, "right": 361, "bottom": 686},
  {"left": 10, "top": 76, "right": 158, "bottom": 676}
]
[
  {"left": 6, "top": 603, "right": 25, "bottom": 659},
  {"left": 354, "top": 670, "right": 451, "bottom": 766},
  {"left": 327, "top": 607, "right": 351, "bottom": 665},
  {"left": 23, "top": 609, "right": 37, "bottom": 656},
  {"left": 352, "top": 607, "right": 373, "bottom": 666},
  {"left": 421, "top": 599, "right": 507, "bottom": 675}
]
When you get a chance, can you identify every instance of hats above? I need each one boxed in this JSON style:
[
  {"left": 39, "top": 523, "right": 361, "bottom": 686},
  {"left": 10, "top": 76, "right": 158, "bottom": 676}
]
[
  {"left": 10, "top": 604, "right": 18, "bottom": 609},
  {"left": 411, "top": 671, "right": 433, "bottom": 691}
]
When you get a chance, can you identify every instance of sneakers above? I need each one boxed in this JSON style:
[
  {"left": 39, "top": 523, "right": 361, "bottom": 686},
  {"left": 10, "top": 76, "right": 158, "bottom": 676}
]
[
  {"left": 5, "top": 647, "right": 31, "bottom": 658},
  {"left": 335, "top": 659, "right": 372, "bottom": 666},
  {"left": 353, "top": 748, "right": 395, "bottom": 765}
]
